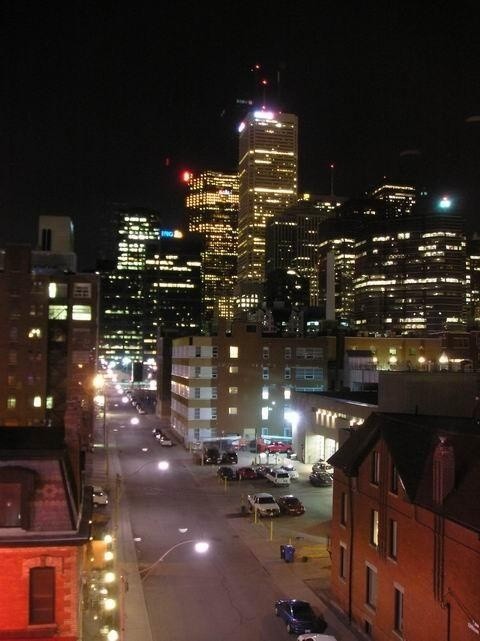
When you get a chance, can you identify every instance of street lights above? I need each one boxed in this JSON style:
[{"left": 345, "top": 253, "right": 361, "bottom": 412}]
[
  {"left": 113, "top": 460, "right": 169, "bottom": 570},
  {"left": 104, "top": 369, "right": 129, "bottom": 491},
  {"left": 119, "top": 540, "right": 210, "bottom": 641}
]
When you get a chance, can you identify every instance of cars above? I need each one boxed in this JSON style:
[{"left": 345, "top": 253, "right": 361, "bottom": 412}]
[
  {"left": 274, "top": 599, "right": 338, "bottom": 641},
  {"left": 92, "top": 486, "right": 109, "bottom": 508},
  {"left": 203, "top": 448, "right": 237, "bottom": 465},
  {"left": 309, "top": 461, "right": 335, "bottom": 487},
  {"left": 125, "top": 388, "right": 147, "bottom": 415},
  {"left": 268, "top": 442, "right": 292, "bottom": 453},
  {"left": 216, "top": 465, "right": 299, "bottom": 487},
  {"left": 275, "top": 495, "right": 305, "bottom": 514},
  {"left": 152, "top": 428, "right": 172, "bottom": 448}
]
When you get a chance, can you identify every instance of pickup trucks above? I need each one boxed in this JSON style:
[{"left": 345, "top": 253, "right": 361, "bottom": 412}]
[{"left": 248, "top": 493, "right": 280, "bottom": 517}]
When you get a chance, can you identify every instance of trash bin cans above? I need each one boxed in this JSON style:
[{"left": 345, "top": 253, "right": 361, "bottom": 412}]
[
  {"left": 92, "top": 541, "right": 107, "bottom": 570},
  {"left": 280, "top": 544, "right": 295, "bottom": 561}
]
